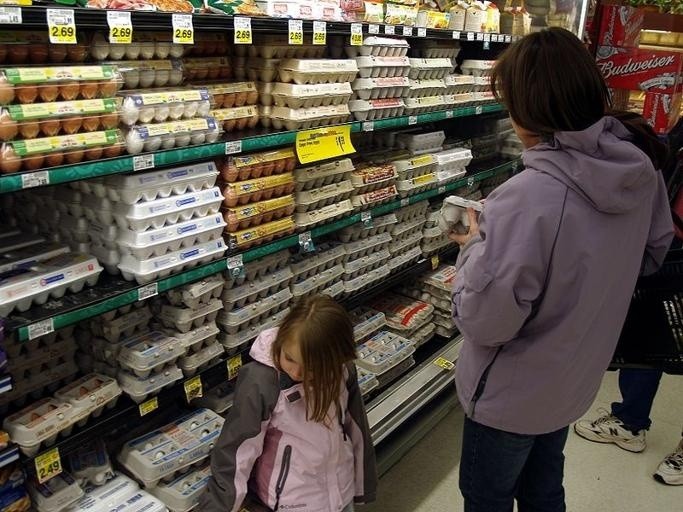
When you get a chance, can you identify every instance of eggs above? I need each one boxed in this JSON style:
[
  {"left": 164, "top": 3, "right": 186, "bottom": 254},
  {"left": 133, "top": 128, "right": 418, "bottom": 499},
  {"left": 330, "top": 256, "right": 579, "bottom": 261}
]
[{"left": 0, "top": 29, "right": 504, "bottom": 512}]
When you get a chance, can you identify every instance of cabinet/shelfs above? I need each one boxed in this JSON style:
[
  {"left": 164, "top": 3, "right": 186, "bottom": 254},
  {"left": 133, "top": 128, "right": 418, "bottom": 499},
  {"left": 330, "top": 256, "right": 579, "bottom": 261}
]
[{"left": 0, "top": 1, "right": 588, "bottom": 511}]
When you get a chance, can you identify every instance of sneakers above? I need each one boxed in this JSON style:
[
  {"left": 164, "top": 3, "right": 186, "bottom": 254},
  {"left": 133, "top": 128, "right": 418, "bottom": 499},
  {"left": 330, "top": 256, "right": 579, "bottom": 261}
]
[{"left": 574, "top": 407, "right": 683, "bottom": 486}]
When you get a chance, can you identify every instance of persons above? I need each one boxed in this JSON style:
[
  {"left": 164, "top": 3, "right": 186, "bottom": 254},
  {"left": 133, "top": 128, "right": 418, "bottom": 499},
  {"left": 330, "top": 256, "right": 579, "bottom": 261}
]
[
  {"left": 448, "top": 24, "right": 677, "bottom": 512},
  {"left": 197, "top": 291, "right": 378, "bottom": 512},
  {"left": 574, "top": 110, "right": 682, "bottom": 488}
]
